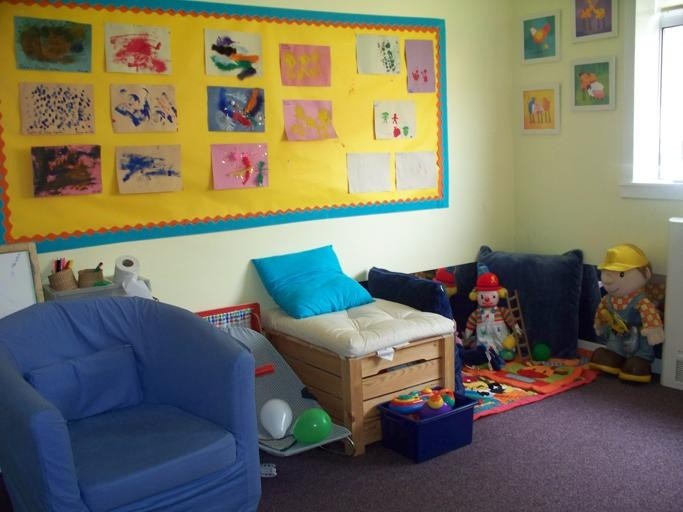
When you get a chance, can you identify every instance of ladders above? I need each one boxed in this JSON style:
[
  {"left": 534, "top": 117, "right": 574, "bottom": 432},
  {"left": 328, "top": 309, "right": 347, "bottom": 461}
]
[{"left": 506, "top": 290, "right": 532, "bottom": 362}]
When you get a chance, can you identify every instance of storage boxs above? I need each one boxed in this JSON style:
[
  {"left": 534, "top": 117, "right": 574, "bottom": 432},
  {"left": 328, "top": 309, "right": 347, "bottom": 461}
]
[
  {"left": 42, "top": 271, "right": 153, "bottom": 300},
  {"left": 374, "top": 385, "right": 478, "bottom": 465}
]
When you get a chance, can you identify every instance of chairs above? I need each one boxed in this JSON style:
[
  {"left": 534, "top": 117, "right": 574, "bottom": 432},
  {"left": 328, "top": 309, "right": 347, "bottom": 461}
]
[{"left": 0, "top": 294, "right": 263, "bottom": 511}]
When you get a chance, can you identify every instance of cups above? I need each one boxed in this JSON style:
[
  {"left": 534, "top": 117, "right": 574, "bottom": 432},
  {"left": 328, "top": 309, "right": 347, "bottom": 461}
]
[
  {"left": 77, "top": 269, "right": 104, "bottom": 288},
  {"left": 47, "top": 269, "right": 79, "bottom": 290}
]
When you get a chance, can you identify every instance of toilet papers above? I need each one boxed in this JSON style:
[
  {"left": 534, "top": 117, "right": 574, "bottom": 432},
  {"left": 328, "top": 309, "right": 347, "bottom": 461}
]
[{"left": 114, "top": 255, "right": 153, "bottom": 300}]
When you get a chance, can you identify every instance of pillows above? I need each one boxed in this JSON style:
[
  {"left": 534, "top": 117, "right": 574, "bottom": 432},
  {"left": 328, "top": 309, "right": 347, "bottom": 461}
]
[
  {"left": 247, "top": 242, "right": 375, "bottom": 319},
  {"left": 366, "top": 265, "right": 468, "bottom": 394},
  {"left": 474, "top": 242, "right": 584, "bottom": 360}
]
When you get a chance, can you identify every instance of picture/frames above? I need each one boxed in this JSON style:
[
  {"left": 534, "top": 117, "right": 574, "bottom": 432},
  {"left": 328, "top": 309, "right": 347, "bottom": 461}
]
[{"left": 517, "top": 0, "right": 618, "bottom": 136}]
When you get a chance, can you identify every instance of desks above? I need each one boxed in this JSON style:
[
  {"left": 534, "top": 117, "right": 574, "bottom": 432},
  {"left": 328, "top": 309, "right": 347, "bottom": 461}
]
[{"left": 263, "top": 327, "right": 456, "bottom": 459}]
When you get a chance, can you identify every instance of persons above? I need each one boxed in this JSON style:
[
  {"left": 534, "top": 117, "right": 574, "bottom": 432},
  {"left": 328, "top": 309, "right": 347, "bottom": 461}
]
[
  {"left": 465, "top": 272, "right": 524, "bottom": 361},
  {"left": 588, "top": 243, "right": 664, "bottom": 383}
]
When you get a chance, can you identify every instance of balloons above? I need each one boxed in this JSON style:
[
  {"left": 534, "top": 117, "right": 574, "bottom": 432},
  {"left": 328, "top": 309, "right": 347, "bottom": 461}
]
[
  {"left": 292, "top": 407, "right": 332, "bottom": 444},
  {"left": 260, "top": 398, "right": 292, "bottom": 439}
]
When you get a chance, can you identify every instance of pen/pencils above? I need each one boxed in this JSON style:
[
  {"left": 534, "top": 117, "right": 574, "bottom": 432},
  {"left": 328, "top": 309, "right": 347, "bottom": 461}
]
[
  {"left": 95, "top": 262, "right": 103, "bottom": 272},
  {"left": 53, "top": 257, "right": 70, "bottom": 274}
]
